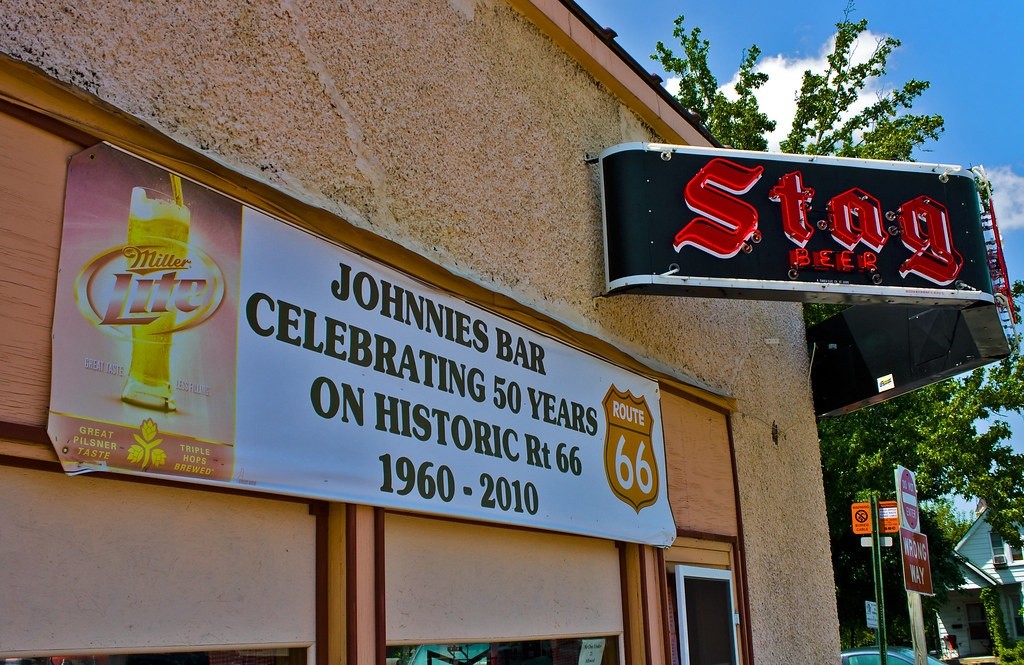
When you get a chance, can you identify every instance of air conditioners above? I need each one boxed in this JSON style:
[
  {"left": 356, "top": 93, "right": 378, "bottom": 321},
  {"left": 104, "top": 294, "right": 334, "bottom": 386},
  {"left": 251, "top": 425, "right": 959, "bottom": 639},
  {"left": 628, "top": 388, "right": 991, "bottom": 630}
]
[{"left": 993, "top": 554, "right": 1007, "bottom": 566}]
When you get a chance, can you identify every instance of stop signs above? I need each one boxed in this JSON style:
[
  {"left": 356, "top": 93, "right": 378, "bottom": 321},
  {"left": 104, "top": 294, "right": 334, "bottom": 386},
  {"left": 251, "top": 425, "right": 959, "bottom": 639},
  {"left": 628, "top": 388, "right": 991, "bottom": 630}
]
[{"left": 893, "top": 463, "right": 921, "bottom": 532}]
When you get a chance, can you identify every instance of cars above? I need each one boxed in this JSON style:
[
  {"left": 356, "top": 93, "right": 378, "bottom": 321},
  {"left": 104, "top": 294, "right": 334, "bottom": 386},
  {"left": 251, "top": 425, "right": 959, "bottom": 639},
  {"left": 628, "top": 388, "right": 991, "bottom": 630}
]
[{"left": 841, "top": 646, "right": 948, "bottom": 665}]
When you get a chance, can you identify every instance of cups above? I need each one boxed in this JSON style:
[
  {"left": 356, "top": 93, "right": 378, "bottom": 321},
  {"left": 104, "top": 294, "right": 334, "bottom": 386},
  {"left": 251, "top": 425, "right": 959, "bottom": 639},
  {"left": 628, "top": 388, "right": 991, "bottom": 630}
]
[{"left": 119, "top": 186, "right": 191, "bottom": 412}]
